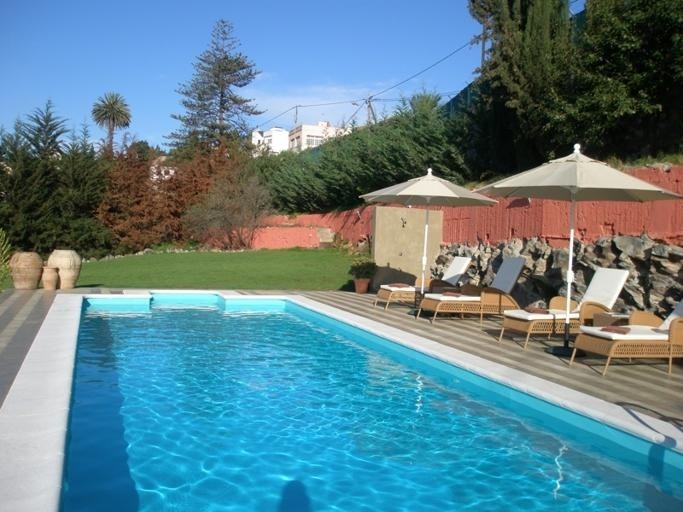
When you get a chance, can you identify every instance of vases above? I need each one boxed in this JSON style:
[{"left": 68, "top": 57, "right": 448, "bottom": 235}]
[{"left": 9, "top": 243, "right": 84, "bottom": 294}]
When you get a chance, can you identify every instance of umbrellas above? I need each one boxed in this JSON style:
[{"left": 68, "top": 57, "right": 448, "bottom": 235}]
[
  {"left": 468, "top": 142, "right": 682, "bottom": 358},
  {"left": 356, "top": 167, "right": 500, "bottom": 317}
]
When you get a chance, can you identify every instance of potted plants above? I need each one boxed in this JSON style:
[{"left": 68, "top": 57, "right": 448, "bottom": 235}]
[{"left": 346, "top": 261, "right": 380, "bottom": 293}]
